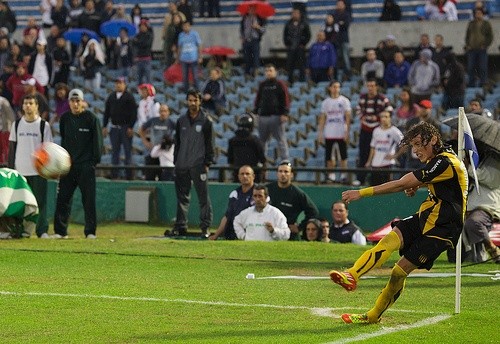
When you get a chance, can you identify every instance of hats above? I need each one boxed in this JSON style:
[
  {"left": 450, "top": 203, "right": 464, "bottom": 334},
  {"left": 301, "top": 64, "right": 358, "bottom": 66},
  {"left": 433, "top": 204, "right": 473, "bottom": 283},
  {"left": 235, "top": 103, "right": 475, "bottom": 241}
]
[
  {"left": 113, "top": 76, "right": 126, "bottom": 82},
  {"left": 68, "top": 89, "right": 84, "bottom": 100},
  {"left": 419, "top": 48, "right": 432, "bottom": 58},
  {"left": 20, "top": 77, "right": 36, "bottom": 86},
  {"left": 416, "top": 100, "right": 432, "bottom": 108},
  {"left": 36, "top": 38, "right": 48, "bottom": 45}
]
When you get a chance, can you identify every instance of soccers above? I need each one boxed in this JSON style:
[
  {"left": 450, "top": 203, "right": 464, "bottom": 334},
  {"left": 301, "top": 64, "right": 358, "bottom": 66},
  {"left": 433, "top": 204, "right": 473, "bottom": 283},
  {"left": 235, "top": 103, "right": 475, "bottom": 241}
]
[{"left": 32, "top": 142, "right": 72, "bottom": 178}]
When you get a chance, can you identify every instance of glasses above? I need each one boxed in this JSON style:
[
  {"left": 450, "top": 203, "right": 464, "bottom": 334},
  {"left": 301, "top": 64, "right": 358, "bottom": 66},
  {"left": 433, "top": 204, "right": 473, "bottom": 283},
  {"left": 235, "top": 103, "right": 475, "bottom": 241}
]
[{"left": 280, "top": 161, "right": 292, "bottom": 167}]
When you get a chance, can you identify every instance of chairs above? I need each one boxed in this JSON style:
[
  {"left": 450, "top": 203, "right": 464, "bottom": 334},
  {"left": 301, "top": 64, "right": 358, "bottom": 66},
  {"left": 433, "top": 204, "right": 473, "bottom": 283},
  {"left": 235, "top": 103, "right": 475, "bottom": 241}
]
[{"left": 50, "top": 62, "right": 500, "bottom": 183}]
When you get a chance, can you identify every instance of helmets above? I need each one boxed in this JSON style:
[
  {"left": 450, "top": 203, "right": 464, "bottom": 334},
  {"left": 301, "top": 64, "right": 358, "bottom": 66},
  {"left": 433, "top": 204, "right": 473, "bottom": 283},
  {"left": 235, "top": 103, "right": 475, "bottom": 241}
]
[
  {"left": 238, "top": 116, "right": 254, "bottom": 132},
  {"left": 137, "top": 83, "right": 155, "bottom": 97}
]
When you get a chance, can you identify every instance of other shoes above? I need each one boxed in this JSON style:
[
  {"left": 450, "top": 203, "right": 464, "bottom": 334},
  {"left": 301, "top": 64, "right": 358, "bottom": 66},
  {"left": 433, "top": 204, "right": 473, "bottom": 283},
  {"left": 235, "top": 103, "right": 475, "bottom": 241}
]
[
  {"left": 86, "top": 234, "right": 96, "bottom": 239},
  {"left": 340, "top": 178, "right": 350, "bottom": 185},
  {"left": 200, "top": 227, "right": 210, "bottom": 239},
  {"left": 164, "top": 227, "right": 188, "bottom": 236},
  {"left": 50, "top": 234, "right": 69, "bottom": 239},
  {"left": 322, "top": 178, "right": 335, "bottom": 184},
  {"left": 487, "top": 246, "right": 500, "bottom": 263},
  {"left": 40, "top": 233, "right": 50, "bottom": 239}
]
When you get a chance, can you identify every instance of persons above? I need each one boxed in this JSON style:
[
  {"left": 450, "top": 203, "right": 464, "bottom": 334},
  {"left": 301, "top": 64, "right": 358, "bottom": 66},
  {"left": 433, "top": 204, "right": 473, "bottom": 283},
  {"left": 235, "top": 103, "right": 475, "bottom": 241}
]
[
  {"left": 329, "top": 121, "right": 469, "bottom": 324},
  {"left": 0, "top": 0, "right": 500, "bottom": 265}
]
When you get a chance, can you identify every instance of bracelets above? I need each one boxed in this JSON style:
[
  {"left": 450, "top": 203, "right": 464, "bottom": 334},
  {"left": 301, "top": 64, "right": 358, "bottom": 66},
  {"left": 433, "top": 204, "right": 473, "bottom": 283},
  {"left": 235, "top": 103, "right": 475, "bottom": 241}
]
[{"left": 359, "top": 186, "right": 374, "bottom": 197}]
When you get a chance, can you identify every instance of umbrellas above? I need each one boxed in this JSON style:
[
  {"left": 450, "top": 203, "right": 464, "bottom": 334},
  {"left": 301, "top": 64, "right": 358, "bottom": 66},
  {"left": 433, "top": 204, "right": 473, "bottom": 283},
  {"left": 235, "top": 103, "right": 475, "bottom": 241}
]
[
  {"left": 100, "top": 20, "right": 136, "bottom": 38},
  {"left": 237, "top": 0, "right": 275, "bottom": 18},
  {"left": 62, "top": 28, "right": 100, "bottom": 45}
]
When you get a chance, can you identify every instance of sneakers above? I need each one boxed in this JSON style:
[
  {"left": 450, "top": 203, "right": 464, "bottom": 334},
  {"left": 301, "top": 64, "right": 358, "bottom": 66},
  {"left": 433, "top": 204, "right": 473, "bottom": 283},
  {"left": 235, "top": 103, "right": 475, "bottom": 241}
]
[
  {"left": 329, "top": 270, "right": 357, "bottom": 292},
  {"left": 341, "top": 312, "right": 381, "bottom": 324}
]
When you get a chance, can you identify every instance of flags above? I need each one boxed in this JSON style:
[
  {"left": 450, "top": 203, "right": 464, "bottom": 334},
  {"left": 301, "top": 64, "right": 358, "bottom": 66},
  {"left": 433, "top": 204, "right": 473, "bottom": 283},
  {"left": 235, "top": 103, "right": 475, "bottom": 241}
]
[{"left": 461, "top": 108, "right": 480, "bottom": 195}]
